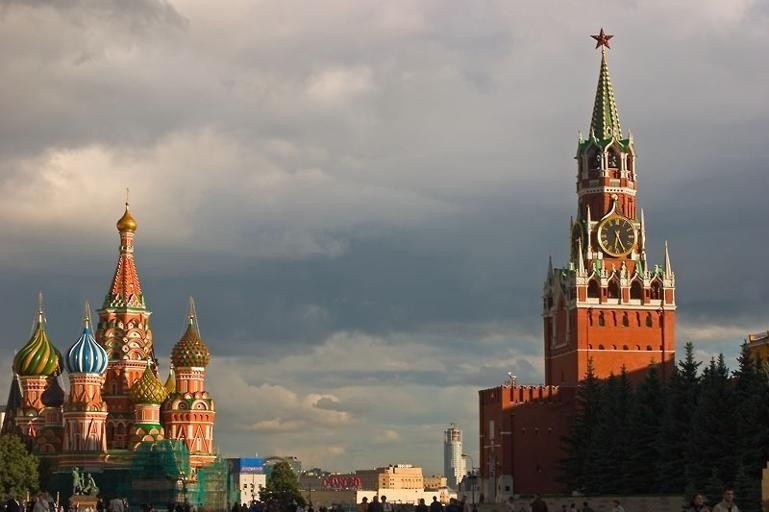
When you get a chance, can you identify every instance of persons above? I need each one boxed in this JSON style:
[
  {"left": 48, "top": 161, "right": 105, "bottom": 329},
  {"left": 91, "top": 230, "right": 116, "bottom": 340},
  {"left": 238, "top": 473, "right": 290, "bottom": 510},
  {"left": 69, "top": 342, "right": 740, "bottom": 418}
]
[
  {"left": 232, "top": 496, "right": 313, "bottom": 511},
  {"left": 175, "top": 497, "right": 205, "bottom": 511},
  {"left": 1, "top": 496, "right": 129, "bottom": 512},
  {"left": 688, "top": 487, "right": 739, "bottom": 512},
  {"left": 505, "top": 497, "right": 624, "bottom": 512},
  {"left": 416, "top": 495, "right": 471, "bottom": 511},
  {"left": 319, "top": 495, "right": 393, "bottom": 511}
]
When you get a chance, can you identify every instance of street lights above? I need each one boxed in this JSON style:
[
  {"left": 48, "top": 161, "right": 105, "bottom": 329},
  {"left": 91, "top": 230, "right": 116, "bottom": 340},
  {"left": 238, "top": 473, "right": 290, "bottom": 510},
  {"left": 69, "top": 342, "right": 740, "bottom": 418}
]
[{"left": 460, "top": 454, "right": 474, "bottom": 505}]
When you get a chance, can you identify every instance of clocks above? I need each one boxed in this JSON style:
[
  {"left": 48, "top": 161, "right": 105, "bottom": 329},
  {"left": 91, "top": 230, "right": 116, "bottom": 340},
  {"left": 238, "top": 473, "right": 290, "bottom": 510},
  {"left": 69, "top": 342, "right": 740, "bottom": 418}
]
[{"left": 597, "top": 215, "right": 638, "bottom": 256}]
